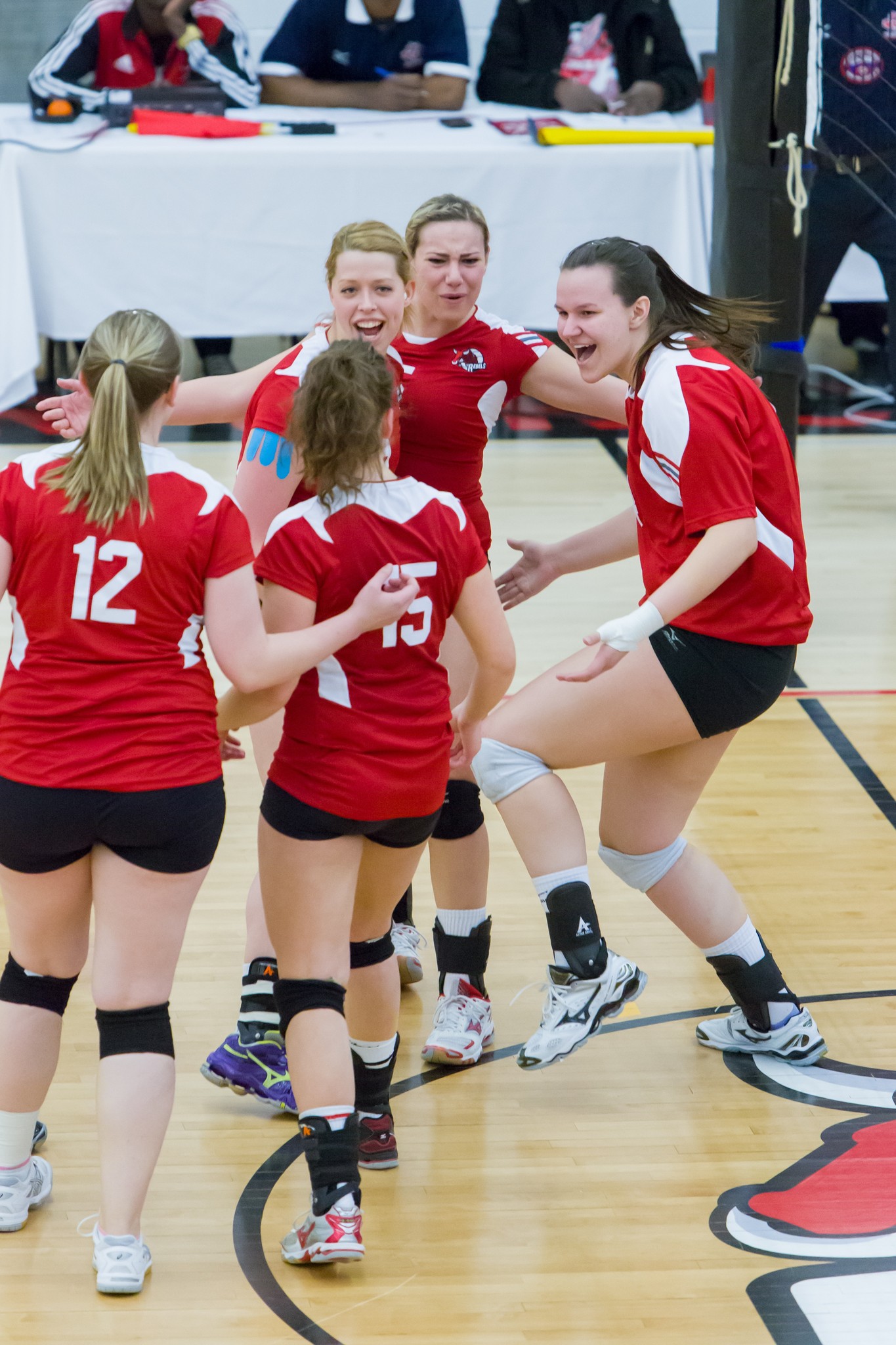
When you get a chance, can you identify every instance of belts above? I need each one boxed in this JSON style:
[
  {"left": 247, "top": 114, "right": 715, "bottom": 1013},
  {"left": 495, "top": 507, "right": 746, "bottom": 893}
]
[{"left": 818, "top": 150, "right": 896, "bottom": 175}]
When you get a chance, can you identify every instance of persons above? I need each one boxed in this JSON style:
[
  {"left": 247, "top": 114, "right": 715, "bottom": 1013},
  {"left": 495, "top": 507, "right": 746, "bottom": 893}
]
[
  {"left": 34, "top": 192, "right": 778, "bottom": 1064},
  {"left": 474, "top": 0, "right": 699, "bottom": 116},
  {"left": 0, "top": 308, "right": 420, "bottom": 1292},
  {"left": 199, "top": 220, "right": 415, "bottom": 1117},
  {"left": 471, "top": 237, "right": 829, "bottom": 1067},
  {"left": 255, "top": 0, "right": 473, "bottom": 110},
  {"left": 213, "top": 341, "right": 515, "bottom": 1266},
  {"left": 25, "top": 0, "right": 260, "bottom": 113}
]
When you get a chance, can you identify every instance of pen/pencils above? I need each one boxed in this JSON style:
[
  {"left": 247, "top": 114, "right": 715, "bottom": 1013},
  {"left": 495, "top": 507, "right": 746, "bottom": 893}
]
[{"left": 374, "top": 66, "right": 431, "bottom": 98}]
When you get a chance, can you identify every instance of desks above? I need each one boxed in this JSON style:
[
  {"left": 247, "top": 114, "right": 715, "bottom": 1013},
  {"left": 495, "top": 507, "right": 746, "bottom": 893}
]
[{"left": 0, "top": 105, "right": 889, "bottom": 413}]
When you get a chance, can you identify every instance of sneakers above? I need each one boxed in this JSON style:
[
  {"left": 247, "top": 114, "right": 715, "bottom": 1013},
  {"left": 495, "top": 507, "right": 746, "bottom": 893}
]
[
  {"left": 0, "top": 1155, "right": 53, "bottom": 1233},
  {"left": 75, "top": 1214, "right": 153, "bottom": 1294},
  {"left": 419, "top": 977, "right": 497, "bottom": 1066},
  {"left": 391, "top": 920, "right": 427, "bottom": 987},
  {"left": 199, "top": 1030, "right": 300, "bottom": 1115},
  {"left": 280, "top": 1206, "right": 366, "bottom": 1265},
  {"left": 515, "top": 939, "right": 646, "bottom": 1069},
  {"left": 695, "top": 1004, "right": 828, "bottom": 1066},
  {"left": 355, "top": 1091, "right": 401, "bottom": 1170}
]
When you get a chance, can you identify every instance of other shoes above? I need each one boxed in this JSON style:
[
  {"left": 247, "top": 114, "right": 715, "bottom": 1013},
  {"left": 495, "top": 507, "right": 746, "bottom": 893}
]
[
  {"left": 32, "top": 1121, "right": 48, "bottom": 1151},
  {"left": 840, "top": 350, "right": 893, "bottom": 397}
]
